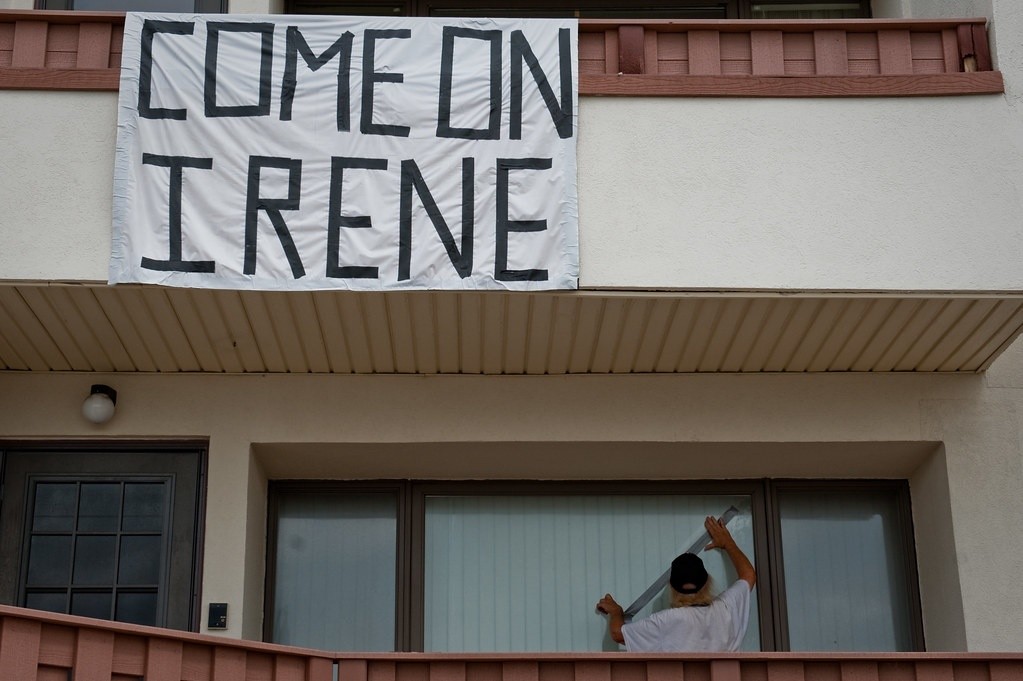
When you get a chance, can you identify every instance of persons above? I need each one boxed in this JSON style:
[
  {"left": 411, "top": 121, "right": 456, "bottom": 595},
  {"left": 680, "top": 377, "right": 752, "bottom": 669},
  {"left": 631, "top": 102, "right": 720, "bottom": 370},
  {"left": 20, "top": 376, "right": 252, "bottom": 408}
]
[{"left": 596, "top": 517, "right": 757, "bottom": 654}]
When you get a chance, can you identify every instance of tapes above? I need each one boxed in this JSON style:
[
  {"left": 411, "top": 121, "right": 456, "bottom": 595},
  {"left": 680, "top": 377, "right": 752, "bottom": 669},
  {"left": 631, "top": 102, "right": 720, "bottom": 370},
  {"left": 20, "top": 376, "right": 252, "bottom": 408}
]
[{"left": 624, "top": 507, "right": 738, "bottom": 626}]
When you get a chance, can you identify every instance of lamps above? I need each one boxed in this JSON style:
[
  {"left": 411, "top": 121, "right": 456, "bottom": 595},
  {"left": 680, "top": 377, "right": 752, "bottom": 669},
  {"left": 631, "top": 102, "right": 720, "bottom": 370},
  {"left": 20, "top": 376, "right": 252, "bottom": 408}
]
[{"left": 80, "top": 383, "right": 118, "bottom": 425}]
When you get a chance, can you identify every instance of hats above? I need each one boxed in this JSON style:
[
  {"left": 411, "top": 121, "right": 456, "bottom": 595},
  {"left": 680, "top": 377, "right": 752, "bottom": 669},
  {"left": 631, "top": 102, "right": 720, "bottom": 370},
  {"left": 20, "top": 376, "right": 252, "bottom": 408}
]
[{"left": 670, "top": 552, "right": 708, "bottom": 594}]
